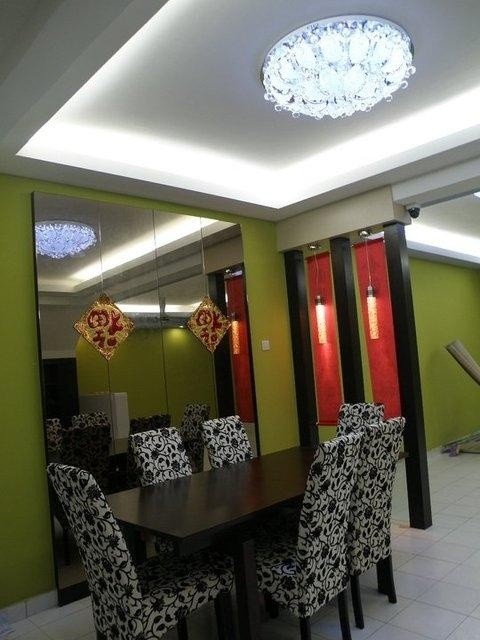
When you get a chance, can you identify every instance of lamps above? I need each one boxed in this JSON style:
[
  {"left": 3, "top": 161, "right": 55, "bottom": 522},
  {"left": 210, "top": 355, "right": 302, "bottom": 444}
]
[
  {"left": 258, "top": 12, "right": 416, "bottom": 126},
  {"left": 306, "top": 244, "right": 331, "bottom": 344},
  {"left": 358, "top": 228, "right": 382, "bottom": 341}
]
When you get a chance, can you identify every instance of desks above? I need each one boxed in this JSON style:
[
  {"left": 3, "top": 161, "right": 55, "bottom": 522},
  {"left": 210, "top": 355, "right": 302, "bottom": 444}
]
[{"left": 100, "top": 443, "right": 412, "bottom": 635}]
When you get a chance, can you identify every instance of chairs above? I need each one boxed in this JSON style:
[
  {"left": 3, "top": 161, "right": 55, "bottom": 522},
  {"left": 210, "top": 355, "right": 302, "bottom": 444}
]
[
  {"left": 330, "top": 401, "right": 389, "bottom": 443},
  {"left": 46, "top": 463, "right": 239, "bottom": 639},
  {"left": 236, "top": 431, "right": 368, "bottom": 639},
  {"left": 200, "top": 413, "right": 254, "bottom": 472},
  {"left": 42, "top": 400, "right": 213, "bottom": 549},
  {"left": 346, "top": 417, "right": 408, "bottom": 630}
]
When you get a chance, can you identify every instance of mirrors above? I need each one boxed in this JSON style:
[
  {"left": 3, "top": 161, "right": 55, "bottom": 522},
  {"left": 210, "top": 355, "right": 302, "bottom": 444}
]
[{"left": 31, "top": 188, "right": 264, "bottom": 611}]
